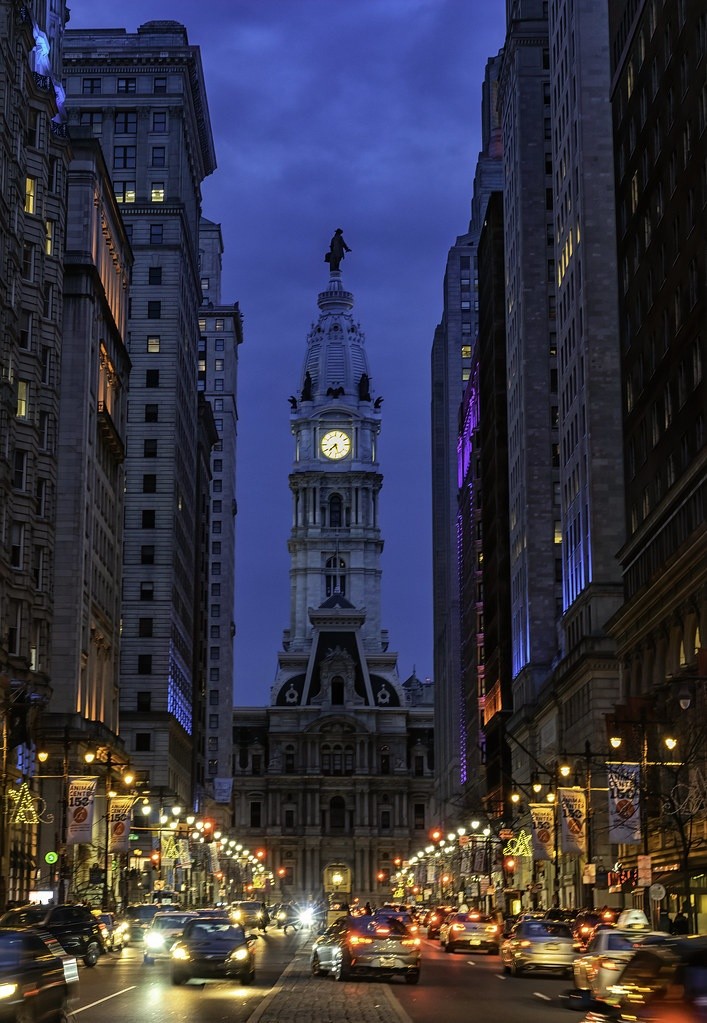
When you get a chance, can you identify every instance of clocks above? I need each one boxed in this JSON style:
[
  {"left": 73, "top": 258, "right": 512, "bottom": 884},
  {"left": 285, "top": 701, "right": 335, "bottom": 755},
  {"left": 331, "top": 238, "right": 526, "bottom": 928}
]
[{"left": 320, "top": 429, "right": 352, "bottom": 460}]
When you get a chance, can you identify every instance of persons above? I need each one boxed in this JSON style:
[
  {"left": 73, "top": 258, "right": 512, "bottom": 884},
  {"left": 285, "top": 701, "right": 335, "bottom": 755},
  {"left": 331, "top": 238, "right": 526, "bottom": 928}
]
[
  {"left": 359, "top": 373, "right": 369, "bottom": 401},
  {"left": 364, "top": 901, "right": 372, "bottom": 916},
  {"left": 284, "top": 908, "right": 298, "bottom": 933},
  {"left": 329, "top": 228, "right": 351, "bottom": 271},
  {"left": 257, "top": 903, "right": 268, "bottom": 933},
  {"left": 301, "top": 371, "right": 312, "bottom": 400}
]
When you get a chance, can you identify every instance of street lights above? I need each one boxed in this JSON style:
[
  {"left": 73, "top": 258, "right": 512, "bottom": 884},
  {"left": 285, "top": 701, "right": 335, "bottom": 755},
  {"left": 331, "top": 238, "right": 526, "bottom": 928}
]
[
  {"left": 396, "top": 761, "right": 562, "bottom": 912},
  {"left": 102, "top": 753, "right": 135, "bottom": 912},
  {"left": 135, "top": 785, "right": 275, "bottom": 903},
  {"left": 38, "top": 734, "right": 96, "bottom": 904},
  {"left": 610, "top": 733, "right": 678, "bottom": 929},
  {"left": 560, "top": 750, "right": 594, "bottom": 907}
]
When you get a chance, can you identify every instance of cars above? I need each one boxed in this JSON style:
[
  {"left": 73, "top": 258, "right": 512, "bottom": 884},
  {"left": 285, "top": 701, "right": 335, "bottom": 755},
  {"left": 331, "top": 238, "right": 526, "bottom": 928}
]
[
  {"left": 121, "top": 888, "right": 320, "bottom": 948},
  {"left": 398, "top": 903, "right": 500, "bottom": 958},
  {"left": 309, "top": 916, "right": 422, "bottom": 984},
  {"left": 498, "top": 921, "right": 587, "bottom": 980},
  {"left": 141, "top": 912, "right": 199, "bottom": 965},
  {"left": 552, "top": 933, "right": 707, "bottom": 1023},
  {"left": 0, "top": 927, "right": 80, "bottom": 1023},
  {"left": 94, "top": 911, "right": 125, "bottom": 953},
  {"left": 374, "top": 908, "right": 419, "bottom": 935},
  {"left": 570, "top": 928, "right": 669, "bottom": 998},
  {"left": 168, "top": 918, "right": 259, "bottom": 986},
  {"left": 502, "top": 907, "right": 623, "bottom": 943},
  {"left": 0, "top": 904, "right": 105, "bottom": 970}
]
